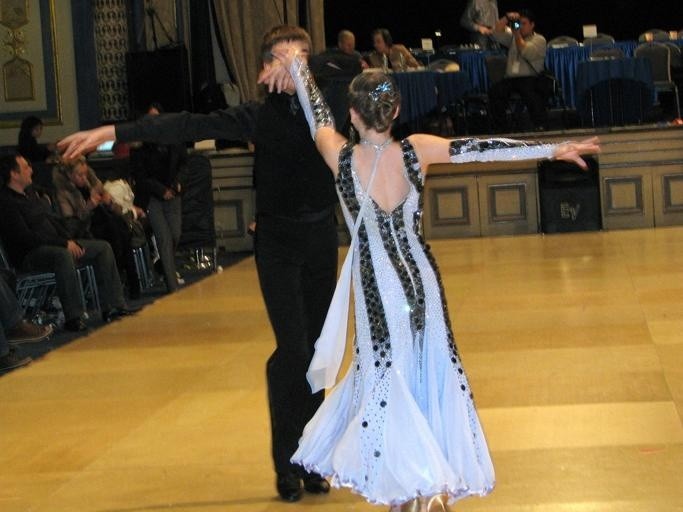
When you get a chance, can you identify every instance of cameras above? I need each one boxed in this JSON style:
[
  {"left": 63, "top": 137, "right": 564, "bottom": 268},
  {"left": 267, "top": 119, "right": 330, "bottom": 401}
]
[{"left": 505, "top": 17, "right": 521, "bottom": 30}]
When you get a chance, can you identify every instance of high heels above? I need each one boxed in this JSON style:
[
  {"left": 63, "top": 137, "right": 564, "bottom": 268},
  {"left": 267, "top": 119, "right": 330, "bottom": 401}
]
[
  {"left": 401, "top": 498, "right": 420, "bottom": 512},
  {"left": 427, "top": 494, "right": 448, "bottom": 512}
]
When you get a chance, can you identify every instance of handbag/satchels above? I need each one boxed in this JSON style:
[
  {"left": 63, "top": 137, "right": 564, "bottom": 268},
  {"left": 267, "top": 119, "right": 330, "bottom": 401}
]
[{"left": 543, "top": 72, "right": 558, "bottom": 96}]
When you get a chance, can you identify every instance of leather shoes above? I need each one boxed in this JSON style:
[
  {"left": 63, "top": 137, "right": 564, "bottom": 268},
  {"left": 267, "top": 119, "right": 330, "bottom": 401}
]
[
  {"left": 8, "top": 319, "right": 52, "bottom": 344},
  {"left": 278, "top": 472, "right": 301, "bottom": 501},
  {"left": 0, "top": 352, "right": 32, "bottom": 370},
  {"left": 65, "top": 317, "right": 86, "bottom": 330},
  {"left": 302, "top": 469, "right": 329, "bottom": 493},
  {"left": 103, "top": 307, "right": 127, "bottom": 318}
]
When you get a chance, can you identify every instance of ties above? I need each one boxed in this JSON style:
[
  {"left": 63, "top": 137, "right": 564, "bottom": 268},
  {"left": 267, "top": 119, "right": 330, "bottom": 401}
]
[{"left": 388, "top": 56, "right": 392, "bottom": 68}]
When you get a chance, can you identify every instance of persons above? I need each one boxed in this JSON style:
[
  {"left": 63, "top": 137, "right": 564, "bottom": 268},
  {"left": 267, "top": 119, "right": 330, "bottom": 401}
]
[
  {"left": 2, "top": 106, "right": 215, "bottom": 368},
  {"left": 56, "top": 27, "right": 362, "bottom": 502},
  {"left": 315, "top": 1, "right": 546, "bottom": 142},
  {"left": 269, "top": 48, "right": 601, "bottom": 512}
]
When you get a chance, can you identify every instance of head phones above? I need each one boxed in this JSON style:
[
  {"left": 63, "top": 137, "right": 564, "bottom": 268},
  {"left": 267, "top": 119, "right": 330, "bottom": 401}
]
[{"left": 382, "top": 26, "right": 393, "bottom": 45}]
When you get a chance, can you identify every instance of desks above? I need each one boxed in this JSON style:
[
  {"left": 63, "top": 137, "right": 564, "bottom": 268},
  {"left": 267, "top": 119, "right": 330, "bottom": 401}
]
[{"left": 319, "top": 39, "right": 683, "bottom": 136}]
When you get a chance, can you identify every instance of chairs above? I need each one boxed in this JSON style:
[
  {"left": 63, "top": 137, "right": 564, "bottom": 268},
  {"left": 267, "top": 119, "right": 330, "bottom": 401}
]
[
  {"left": 0, "top": 248, "right": 103, "bottom": 332},
  {"left": 132, "top": 246, "right": 149, "bottom": 293}
]
